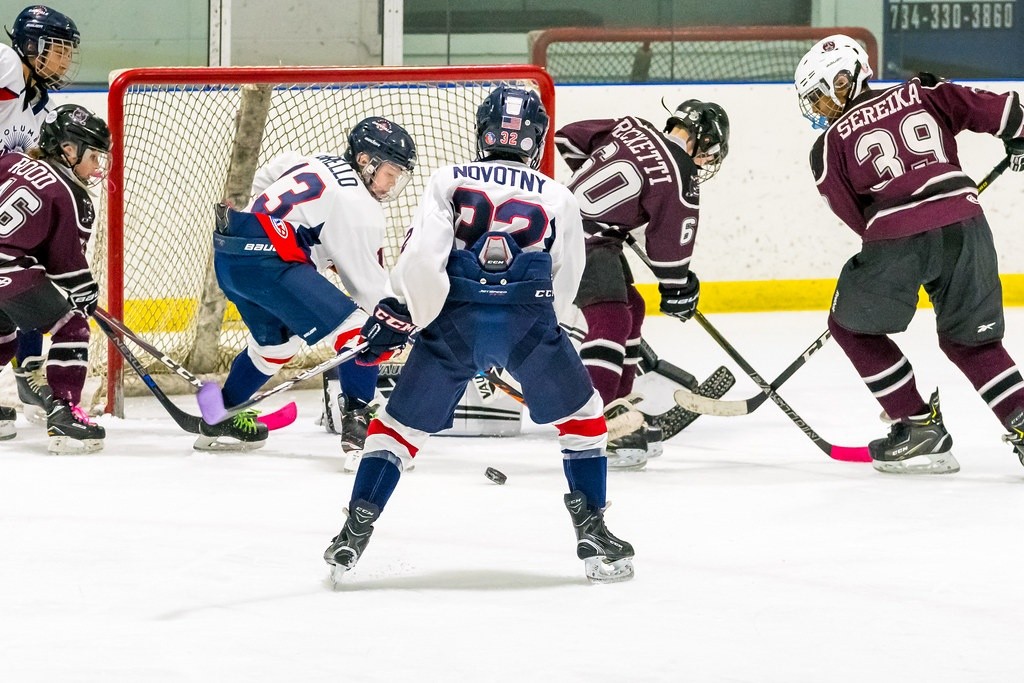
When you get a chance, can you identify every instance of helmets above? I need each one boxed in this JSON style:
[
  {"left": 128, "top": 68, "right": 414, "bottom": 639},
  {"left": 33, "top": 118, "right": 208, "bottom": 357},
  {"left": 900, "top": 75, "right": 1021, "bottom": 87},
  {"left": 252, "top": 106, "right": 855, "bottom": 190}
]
[
  {"left": 39, "top": 104, "right": 114, "bottom": 188},
  {"left": 795, "top": 34, "right": 875, "bottom": 131},
  {"left": 665, "top": 99, "right": 731, "bottom": 186},
  {"left": 474, "top": 86, "right": 551, "bottom": 170},
  {"left": 345, "top": 116, "right": 417, "bottom": 183},
  {"left": 11, "top": 5, "right": 80, "bottom": 91}
]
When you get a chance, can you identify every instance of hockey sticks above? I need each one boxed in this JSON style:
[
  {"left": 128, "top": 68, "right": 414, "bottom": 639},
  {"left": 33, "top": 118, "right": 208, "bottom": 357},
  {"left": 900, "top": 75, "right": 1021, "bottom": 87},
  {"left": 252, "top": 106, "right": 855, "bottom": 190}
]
[
  {"left": 482, "top": 365, "right": 736, "bottom": 441},
  {"left": 673, "top": 154, "right": 1012, "bottom": 417},
  {"left": 94, "top": 304, "right": 298, "bottom": 430},
  {"left": 91, "top": 312, "right": 203, "bottom": 434},
  {"left": 622, "top": 231, "right": 874, "bottom": 463},
  {"left": 195, "top": 342, "right": 368, "bottom": 426}
]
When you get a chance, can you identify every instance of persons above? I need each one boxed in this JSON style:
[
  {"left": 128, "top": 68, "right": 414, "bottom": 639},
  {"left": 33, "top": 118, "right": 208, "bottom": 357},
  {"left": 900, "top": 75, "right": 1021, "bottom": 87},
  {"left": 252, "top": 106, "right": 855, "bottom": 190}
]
[
  {"left": 324, "top": 86, "right": 635, "bottom": 584},
  {"left": 192, "top": 114, "right": 419, "bottom": 472},
  {"left": 0, "top": 5, "right": 84, "bottom": 438},
  {"left": 555, "top": 99, "right": 731, "bottom": 469},
  {"left": 0, "top": 104, "right": 114, "bottom": 456},
  {"left": 794, "top": 33, "right": 1024, "bottom": 474}
]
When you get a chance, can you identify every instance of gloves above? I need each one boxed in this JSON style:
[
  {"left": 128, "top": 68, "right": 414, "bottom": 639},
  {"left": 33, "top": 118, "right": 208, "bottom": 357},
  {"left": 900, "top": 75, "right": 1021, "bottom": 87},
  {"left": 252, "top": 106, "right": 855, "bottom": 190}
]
[
  {"left": 66, "top": 281, "right": 100, "bottom": 317},
  {"left": 1000, "top": 115, "right": 1024, "bottom": 171},
  {"left": 353, "top": 297, "right": 415, "bottom": 366},
  {"left": 658, "top": 271, "right": 700, "bottom": 323}
]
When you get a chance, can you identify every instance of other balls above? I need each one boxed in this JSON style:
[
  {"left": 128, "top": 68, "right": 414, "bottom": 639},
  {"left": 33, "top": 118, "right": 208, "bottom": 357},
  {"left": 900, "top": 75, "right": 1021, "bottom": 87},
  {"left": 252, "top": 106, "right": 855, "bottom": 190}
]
[{"left": 485, "top": 467, "right": 507, "bottom": 485}]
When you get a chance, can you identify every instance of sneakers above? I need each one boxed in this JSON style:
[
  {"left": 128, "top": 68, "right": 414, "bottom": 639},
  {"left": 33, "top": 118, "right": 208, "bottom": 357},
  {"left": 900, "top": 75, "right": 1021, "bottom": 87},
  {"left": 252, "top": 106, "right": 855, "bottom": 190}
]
[
  {"left": 194, "top": 405, "right": 271, "bottom": 451},
  {"left": 321, "top": 365, "right": 344, "bottom": 434},
  {"left": 38, "top": 385, "right": 106, "bottom": 455},
  {"left": 563, "top": 490, "right": 635, "bottom": 579},
  {"left": 0, "top": 405, "right": 17, "bottom": 440},
  {"left": 11, "top": 354, "right": 54, "bottom": 425},
  {"left": 324, "top": 498, "right": 382, "bottom": 588},
  {"left": 335, "top": 392, "right": 414, "bottom": 474},
  {"left": 634, "top": 424, "right": 664, "bottom": 457},
  {"left": 606, "top": 428, "right": 648, "bottom": 467},
  {"left": 868, "top": 386, "right": 960, "bottom": 474},
  {"left": 1001, "top": 409, "right": 1024, "bottom": 469}
]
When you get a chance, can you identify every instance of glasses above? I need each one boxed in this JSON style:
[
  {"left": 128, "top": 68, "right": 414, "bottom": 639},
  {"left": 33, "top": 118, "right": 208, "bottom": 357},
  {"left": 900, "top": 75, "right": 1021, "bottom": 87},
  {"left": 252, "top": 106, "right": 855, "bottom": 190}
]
[{"left": 809, "top": 84, "right": 830, "bottom": 107}]
